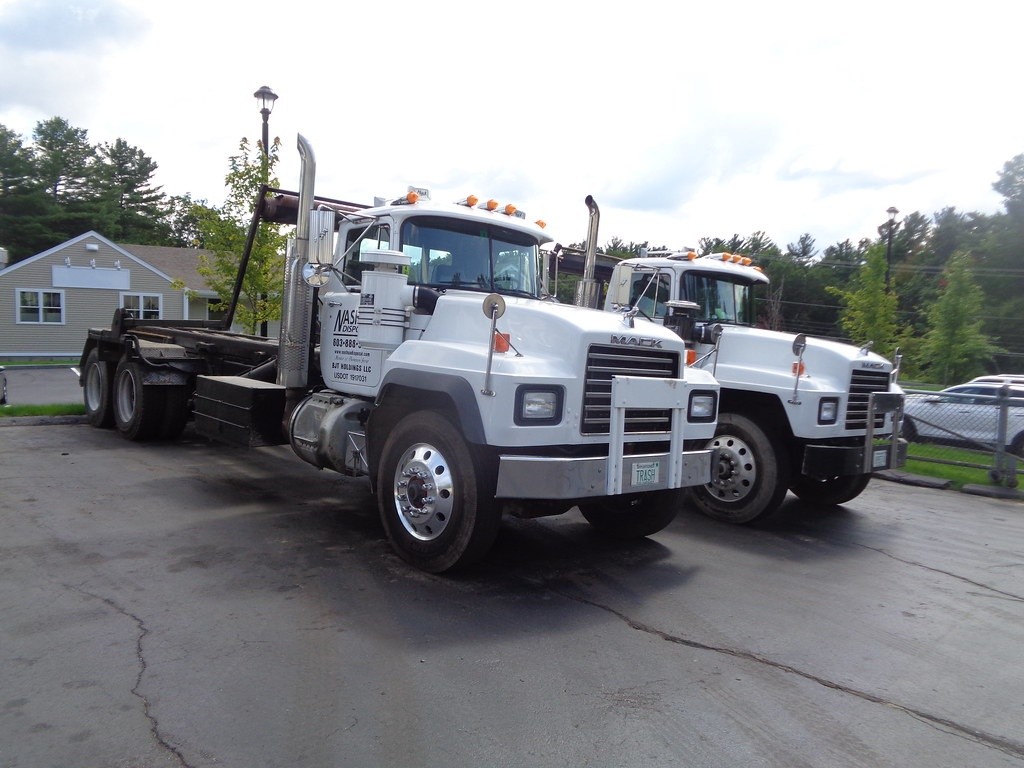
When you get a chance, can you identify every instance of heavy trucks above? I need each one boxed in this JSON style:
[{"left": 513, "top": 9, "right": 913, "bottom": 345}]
[
  {"left": 68, "top": 132, "right": 719, "bottom": 576},
  {"left": 537, "top": 193, "right": 906, "bottom": 527}
]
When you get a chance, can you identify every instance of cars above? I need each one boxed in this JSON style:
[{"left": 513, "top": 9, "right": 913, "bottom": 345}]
[{"left": 901, "top": 372, "right": 1024, "bottom": 458}]
